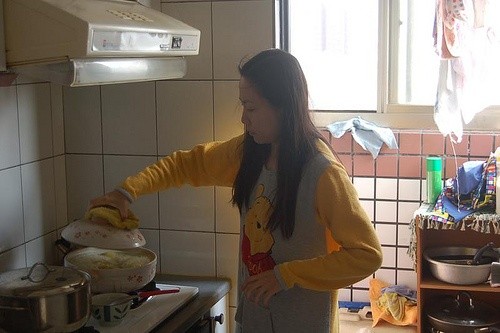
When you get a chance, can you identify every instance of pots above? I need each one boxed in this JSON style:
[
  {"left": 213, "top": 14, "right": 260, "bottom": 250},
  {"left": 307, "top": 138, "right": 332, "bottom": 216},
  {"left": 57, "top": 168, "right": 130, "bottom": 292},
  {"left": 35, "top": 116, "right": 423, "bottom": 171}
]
[
  {"left": 0, "top": 263, "right": 91, "bottom": 333},
  {"left": 64, "top": 246, "right": 157, "bottom": 295},
  {"left": 424, "top": 290, "right": 500, "bottom": 333},
  {"left": 430, "top": 241, "right": 496, "bottom": 265}
]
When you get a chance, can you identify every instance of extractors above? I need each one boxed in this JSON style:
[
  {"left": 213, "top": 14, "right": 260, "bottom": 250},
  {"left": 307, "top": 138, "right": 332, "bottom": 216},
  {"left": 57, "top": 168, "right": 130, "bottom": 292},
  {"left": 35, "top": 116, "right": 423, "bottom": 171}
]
[{"left": 1, "top": 0, "right": 202, "bottom": 86}]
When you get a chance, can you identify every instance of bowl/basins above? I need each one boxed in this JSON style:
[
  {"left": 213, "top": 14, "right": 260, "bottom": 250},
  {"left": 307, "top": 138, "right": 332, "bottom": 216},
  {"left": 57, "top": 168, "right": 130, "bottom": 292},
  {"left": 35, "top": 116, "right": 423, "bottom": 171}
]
[
  {"left": 89, "top": 292, "right": 133, "bottom": 325},
  {"left": 423, "top": 246, "right": 500, "bottom": 286}
]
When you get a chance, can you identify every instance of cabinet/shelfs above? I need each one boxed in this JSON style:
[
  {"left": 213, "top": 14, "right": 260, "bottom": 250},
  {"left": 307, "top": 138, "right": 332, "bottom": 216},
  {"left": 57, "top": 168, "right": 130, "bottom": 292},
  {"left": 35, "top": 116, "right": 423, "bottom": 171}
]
[{"left": 415, "top": 199, "right": 500, "bottom": 333}]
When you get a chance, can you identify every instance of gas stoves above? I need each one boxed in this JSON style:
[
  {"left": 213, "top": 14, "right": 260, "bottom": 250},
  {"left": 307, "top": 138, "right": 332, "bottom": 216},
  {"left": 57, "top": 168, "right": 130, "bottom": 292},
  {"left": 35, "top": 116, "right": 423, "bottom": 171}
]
[{"left": 68, "top": 282, "right": 200, "bottom": 333}]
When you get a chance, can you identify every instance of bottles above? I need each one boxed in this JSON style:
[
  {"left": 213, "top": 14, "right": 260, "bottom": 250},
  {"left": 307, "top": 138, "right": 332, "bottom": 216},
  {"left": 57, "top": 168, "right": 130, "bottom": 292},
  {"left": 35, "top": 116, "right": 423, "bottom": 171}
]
[{"left": 425, "top": 156, "right": 443, "bottom": 205}]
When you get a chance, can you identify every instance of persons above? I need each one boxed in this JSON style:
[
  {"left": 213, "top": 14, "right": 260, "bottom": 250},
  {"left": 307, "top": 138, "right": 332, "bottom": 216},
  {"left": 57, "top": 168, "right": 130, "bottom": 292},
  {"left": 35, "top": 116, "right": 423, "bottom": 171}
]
[{"left": 85, "top": 48, "right": 383, "bottom": 333}]
